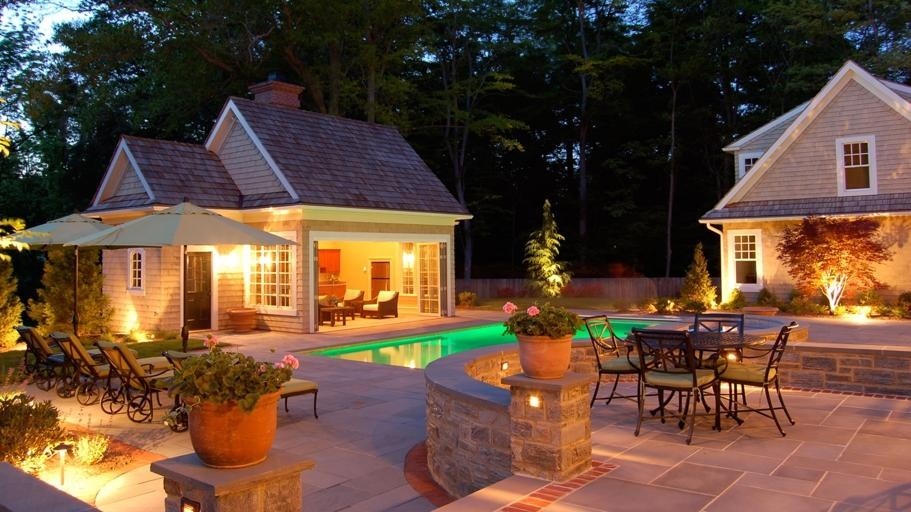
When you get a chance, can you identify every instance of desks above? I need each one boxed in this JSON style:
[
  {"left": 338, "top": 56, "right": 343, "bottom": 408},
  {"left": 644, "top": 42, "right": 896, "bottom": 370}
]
[
  {"left": 319, "top": 307, "right": 346, "bottom": 327},
  {"left": 624, "top": 330, "right": 767, "bottom": 421}
]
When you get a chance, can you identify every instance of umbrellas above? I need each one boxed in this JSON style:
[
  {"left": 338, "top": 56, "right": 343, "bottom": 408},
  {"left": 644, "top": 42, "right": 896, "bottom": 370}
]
[
  {"left": 63, "top": 203, "right": 301, "bottom": 353},
  {"left": 0, "top": 214, "right": 115, "bottom": 338}
]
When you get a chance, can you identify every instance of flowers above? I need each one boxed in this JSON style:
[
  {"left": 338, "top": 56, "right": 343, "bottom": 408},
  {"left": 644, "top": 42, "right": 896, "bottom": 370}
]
[
  {"left": 156, "top": 334, "right": 299, "bottom": 414},
  {"left": 500, "top": 302, "right": 583, "bottom": 340}
]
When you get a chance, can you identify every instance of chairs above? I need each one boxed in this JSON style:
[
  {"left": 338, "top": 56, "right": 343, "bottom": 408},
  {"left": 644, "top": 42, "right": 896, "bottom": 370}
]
[
  {"left": 360, "top": 289, "right": 399, "bottom": 319},
  {"left": 52, "top": 334, "right": 118, "bottom": 406},
  {"left": 581, "top": 315, "right": 657, "bottom": 418},
  {"left": 12, "top": 326, "right": 60, "bottom": 391},
  {"left": 713, "top": 321, "right": 800, "bottom": 437},
  {"left": 163, "top": 349, "right": 318, "bottom": 429},
  {"left": 693, "top": 312, "right": 745, "bottom": 413},
  {"left": 337, "top": 288, "right": 363, "bottom": 314},
  {"left": 93, "top": 342, "right": 176, "bottom": 424},
  {"left": 631, "top": 327, "right": 718, "bottom": 446}
]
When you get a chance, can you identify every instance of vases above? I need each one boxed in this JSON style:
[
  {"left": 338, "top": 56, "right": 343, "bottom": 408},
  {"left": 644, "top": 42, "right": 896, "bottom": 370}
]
[
  {"left": 512, "top": 334, "right": 573, "bottom": 379},
  {"left": 190, "top": 388, "right": 281, "bottom": 468}
]
